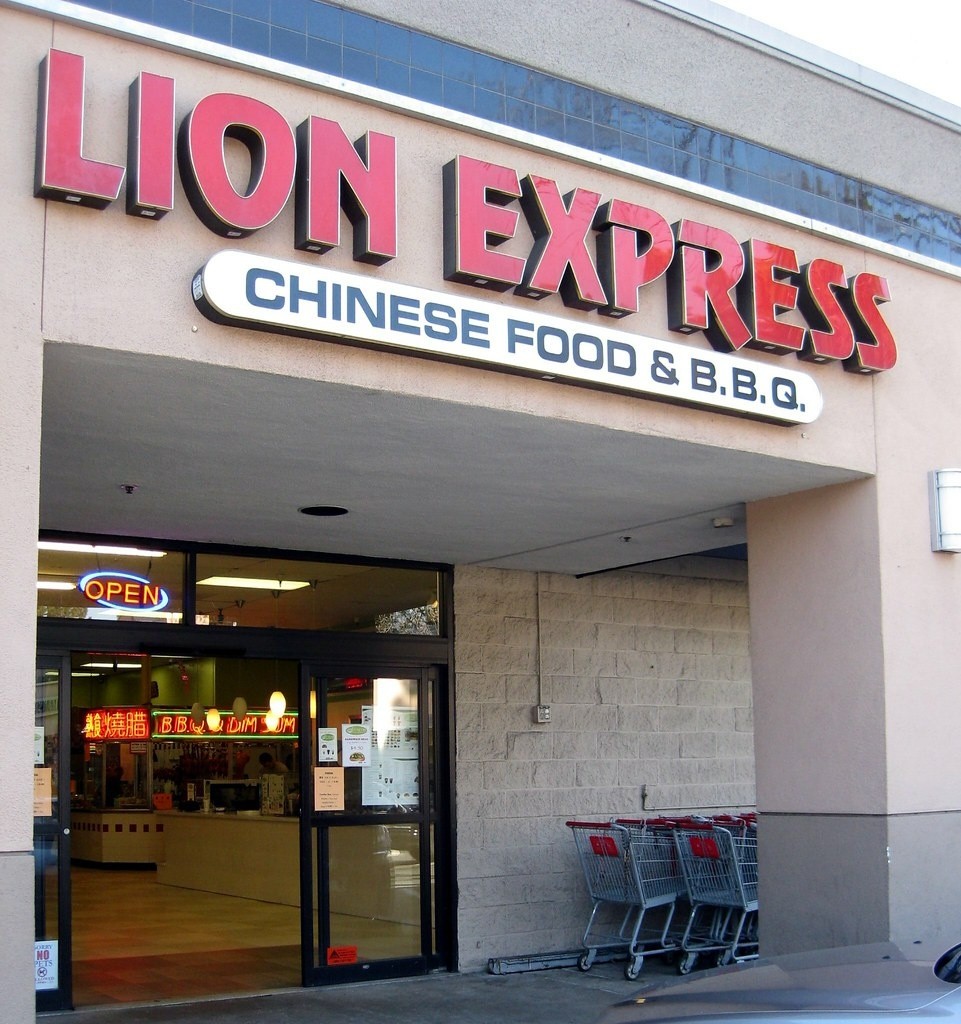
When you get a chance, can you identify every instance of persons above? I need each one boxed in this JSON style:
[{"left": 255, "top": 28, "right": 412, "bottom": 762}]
[{"left": 101, "top": 753, "right": 290, "bottom": 811}]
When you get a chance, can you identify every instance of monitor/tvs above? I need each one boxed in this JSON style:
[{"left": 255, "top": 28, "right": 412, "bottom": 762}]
[{"left": 210, "top": 783, "right": 260, "bottom": 810}]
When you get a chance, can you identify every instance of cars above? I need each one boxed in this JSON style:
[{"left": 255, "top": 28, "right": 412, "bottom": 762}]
[{"left": 589, "top": 935, "right": 961, "bottom": 1024}]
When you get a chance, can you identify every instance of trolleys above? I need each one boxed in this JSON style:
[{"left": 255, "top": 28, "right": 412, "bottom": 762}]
[{"left": 565, "top": 812, "right": 759, "bottom": 982}]
